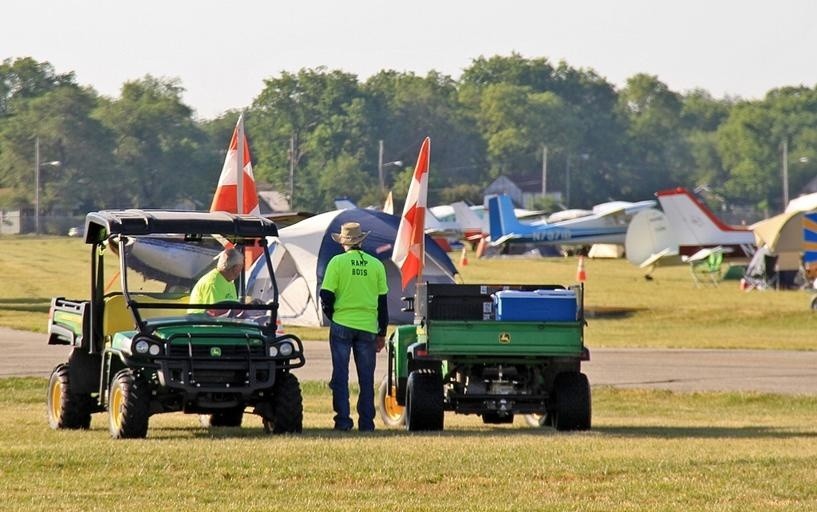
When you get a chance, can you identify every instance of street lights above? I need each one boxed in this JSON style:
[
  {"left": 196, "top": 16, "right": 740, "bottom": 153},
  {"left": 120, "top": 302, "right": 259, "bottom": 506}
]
[
  {"left": 33, "top": 136, "right": 61, "bottom": 231},
  {"left": 780, "top": 138, "right": 810, "bottom": 215},
  {"left": 377, "top": 141, "right": 402, "bottom": 193},
  {"left": 565, "top": 153, "right": 594, "bottom": 210}
]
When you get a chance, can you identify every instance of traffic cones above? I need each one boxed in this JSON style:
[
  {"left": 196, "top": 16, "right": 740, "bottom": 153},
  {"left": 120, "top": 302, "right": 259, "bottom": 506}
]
[{"left": 574, "top": 256, "right": 588, "bottom": 282}]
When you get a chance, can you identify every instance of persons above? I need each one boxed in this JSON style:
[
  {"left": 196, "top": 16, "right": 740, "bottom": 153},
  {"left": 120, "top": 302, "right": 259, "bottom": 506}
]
[
  {"left": 319, "top": 223, "right": 389, "bottom": 432},
  {"left": 187, "top": 247, "right": 267, "bottom": 328}
]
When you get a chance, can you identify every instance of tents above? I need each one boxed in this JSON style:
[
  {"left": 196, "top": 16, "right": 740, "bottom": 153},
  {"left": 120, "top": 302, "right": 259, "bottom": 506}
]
[{"left": 231, "top": 206, "right": 463, "bottom": 327}]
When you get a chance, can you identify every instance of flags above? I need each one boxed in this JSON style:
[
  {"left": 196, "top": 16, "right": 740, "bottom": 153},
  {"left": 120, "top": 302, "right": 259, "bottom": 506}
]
[
  {"left": 390, "top": 136, "right": 429, "bottom": 291},
  {"left": 208, "top": 114, "right": 265, "bottom": 270}
]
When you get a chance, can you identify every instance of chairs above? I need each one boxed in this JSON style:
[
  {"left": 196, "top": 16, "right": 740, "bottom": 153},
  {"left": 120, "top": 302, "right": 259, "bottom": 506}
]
[{"left": 687, "top": 248, "right": 783, "bottom": 295}]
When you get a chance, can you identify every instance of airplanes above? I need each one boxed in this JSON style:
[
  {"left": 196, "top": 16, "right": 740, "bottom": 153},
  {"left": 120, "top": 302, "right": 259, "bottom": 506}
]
[
  {"left": 482, "top": 193, "right": 658, "bottom": 247},
  {"left": 451, "top": 200, "right": 592, "bottom": 242},
  {"left": 423, "top": 207, "right": 544, "bottom": 235}
]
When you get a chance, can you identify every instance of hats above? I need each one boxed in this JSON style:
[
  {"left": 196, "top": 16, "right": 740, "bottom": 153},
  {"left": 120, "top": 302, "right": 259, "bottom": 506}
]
[{"left": 332, "top": 222, "right": 372, "bottom": 246}]
